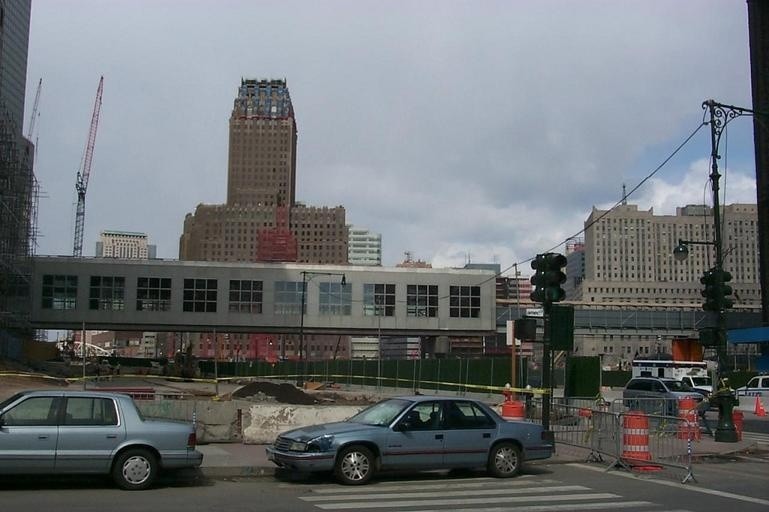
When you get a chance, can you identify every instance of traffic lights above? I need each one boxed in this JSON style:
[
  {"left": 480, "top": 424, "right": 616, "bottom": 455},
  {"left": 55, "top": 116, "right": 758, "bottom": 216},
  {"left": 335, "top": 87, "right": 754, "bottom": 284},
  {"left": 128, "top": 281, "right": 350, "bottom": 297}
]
[
  {"left": 699, "top": 269, "right": 719, "bottom": 311},
  {"left": 719, "top": 268, "right": 733, "bottom": 308},
  {"left": 544, "top": 251, "right": 566, "bottom": 303},
  {"left": 531, "top": 253, "right": 544, "bottom": 303}
]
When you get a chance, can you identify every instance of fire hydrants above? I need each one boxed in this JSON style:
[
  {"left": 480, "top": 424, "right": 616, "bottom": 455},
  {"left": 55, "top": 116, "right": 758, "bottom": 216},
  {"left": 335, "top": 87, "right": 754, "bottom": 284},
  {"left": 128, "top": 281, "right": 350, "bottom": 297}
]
[{"left": 732, "top": 409, "right": 744, "bottom": 442}]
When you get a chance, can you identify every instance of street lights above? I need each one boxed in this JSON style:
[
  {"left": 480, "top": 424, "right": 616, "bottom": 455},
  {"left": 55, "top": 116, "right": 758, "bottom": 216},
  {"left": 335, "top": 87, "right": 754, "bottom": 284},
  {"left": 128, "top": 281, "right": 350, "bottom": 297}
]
[
  {"left": 298, "top": 269, "right": 348, "bottom": 388},
  {"left": 673, "top": 238, "right": 741, "bottom": 442},
  {"left": 253, "top": 335, "right": 273, "bottom": 377}
]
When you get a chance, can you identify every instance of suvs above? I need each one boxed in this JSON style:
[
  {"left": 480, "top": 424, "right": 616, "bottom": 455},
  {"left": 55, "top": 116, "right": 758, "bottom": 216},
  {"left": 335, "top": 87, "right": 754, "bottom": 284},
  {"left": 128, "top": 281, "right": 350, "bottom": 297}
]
[
  {"left": 624, "top": 377, "right": 705, "bottom": 418},
  {"left": 734, "top": 374, "right": 769, "bottom": 399}
]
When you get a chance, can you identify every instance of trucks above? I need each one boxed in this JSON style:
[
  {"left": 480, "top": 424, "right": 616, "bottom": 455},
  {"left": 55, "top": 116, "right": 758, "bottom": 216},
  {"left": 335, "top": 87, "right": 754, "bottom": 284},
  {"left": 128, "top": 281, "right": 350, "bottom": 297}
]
[{"left": 630, "top": 359, "right": 713, "bottom": 398}]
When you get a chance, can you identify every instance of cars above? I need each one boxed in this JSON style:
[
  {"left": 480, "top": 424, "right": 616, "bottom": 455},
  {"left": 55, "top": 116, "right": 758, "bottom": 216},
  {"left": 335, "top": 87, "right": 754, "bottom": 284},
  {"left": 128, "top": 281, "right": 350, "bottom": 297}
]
[
  {"left": 263, "top": 393, "right": 556, "bottom": 487},
  {"left": 0, "top": 389, "right": 205, "bottom": 491}
]
